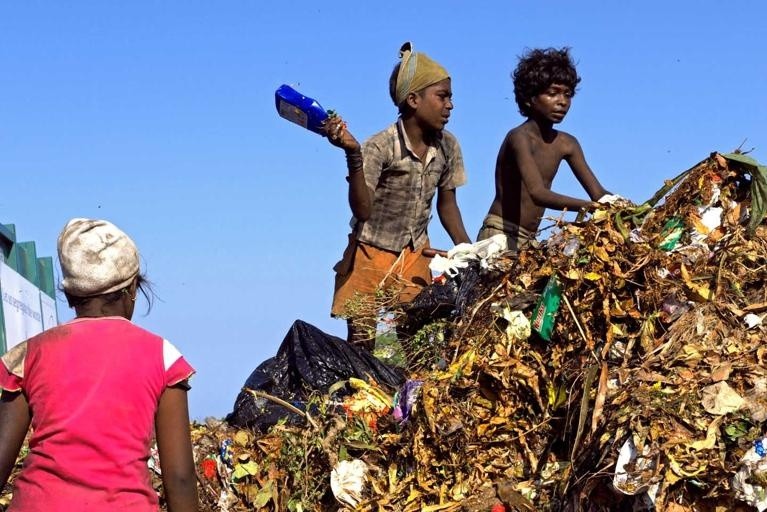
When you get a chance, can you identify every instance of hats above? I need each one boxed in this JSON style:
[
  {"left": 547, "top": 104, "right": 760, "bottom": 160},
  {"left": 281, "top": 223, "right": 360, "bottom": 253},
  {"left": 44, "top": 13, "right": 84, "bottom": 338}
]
[{"left": 57, "top": 218, "right": 140, "bottom": 298}]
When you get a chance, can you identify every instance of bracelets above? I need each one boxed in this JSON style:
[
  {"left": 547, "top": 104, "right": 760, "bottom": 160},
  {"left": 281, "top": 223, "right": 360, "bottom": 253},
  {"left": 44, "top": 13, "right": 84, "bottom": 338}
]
[{"left": 345, "top": 152, "right": 363, "bottom": 184}]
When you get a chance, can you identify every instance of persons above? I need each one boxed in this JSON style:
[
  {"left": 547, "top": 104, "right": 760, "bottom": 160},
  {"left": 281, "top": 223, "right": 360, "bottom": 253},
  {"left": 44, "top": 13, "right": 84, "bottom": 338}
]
[
  {"left": 476, "top": 47, "right": 636, "bottom": 251},
  {"left": 0, "top": 216, "right": 198, "bottom": 512},
  {"left": 321, "top": 40, "right": 472, "bottom": 357}
]
